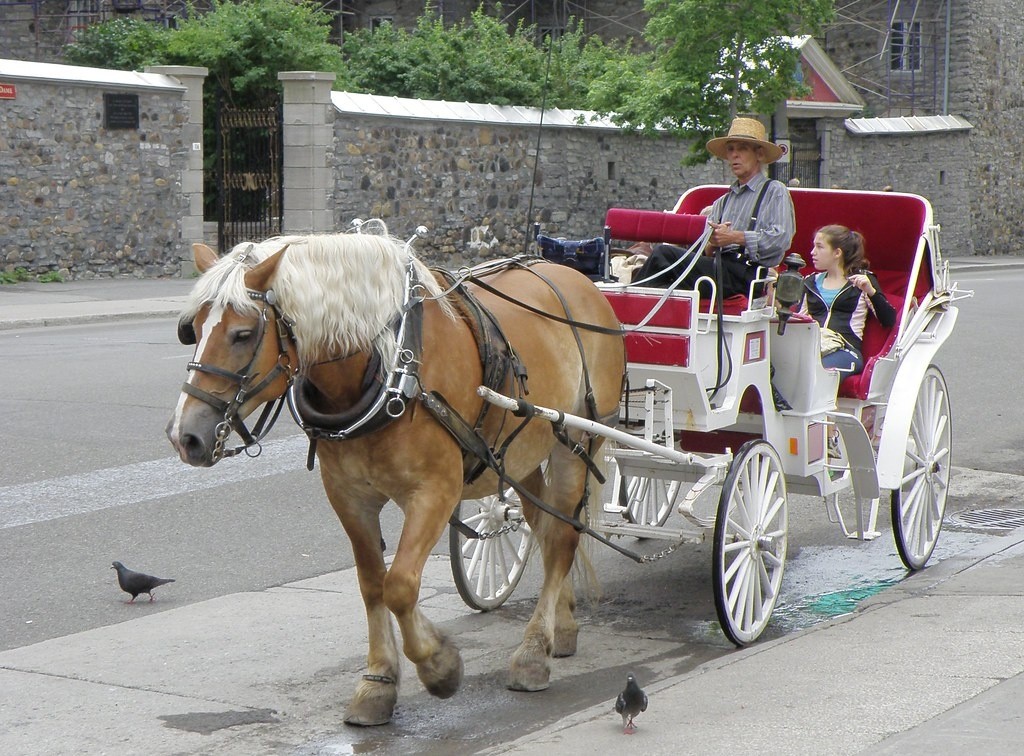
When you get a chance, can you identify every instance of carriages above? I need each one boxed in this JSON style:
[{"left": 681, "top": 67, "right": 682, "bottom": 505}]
[{"left": 165, "top": 182, "right": 977, "bottom": 728}]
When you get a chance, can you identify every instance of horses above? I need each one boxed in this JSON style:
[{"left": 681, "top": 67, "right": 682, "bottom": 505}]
[{"left": 166, "top": 218, "right": 624, "bottom": 726}]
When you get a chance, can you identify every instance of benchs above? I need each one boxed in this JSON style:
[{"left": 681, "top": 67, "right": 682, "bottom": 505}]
[{"left": 593, "top": 181, "right": 939, "bottom": 459}]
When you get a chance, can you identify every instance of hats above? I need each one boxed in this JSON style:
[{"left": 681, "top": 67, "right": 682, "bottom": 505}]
[{"left": 706, "top": 118, "right": 783, "bottom": 165}]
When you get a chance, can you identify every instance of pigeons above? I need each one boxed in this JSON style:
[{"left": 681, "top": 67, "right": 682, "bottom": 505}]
[
  {"left": 110, "top": 561, "right": 175, "bottom": 604},
  {"left": 616, "top": 673, "right": 648, "bottom": 734}
]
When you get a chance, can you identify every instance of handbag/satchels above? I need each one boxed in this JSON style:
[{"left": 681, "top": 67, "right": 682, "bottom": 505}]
[
  {"left": 819, "top": 327, "right": 845, "bottom": 357},
  {"left": 537, "top": 233, "right": 613, "bottom": 283}
]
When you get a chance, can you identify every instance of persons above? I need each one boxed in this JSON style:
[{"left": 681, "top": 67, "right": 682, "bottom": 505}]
[
  {"left": 795, "top": 224, "right": 897, "bottom": 386},
  {"left": 631, "top": 118, "right": 796, "bottom": 300}
]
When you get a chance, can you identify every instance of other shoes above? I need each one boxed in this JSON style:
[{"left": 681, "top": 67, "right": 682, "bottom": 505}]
[{"left": 826, "top": 429, "right": 843, "bottom": 459}]
